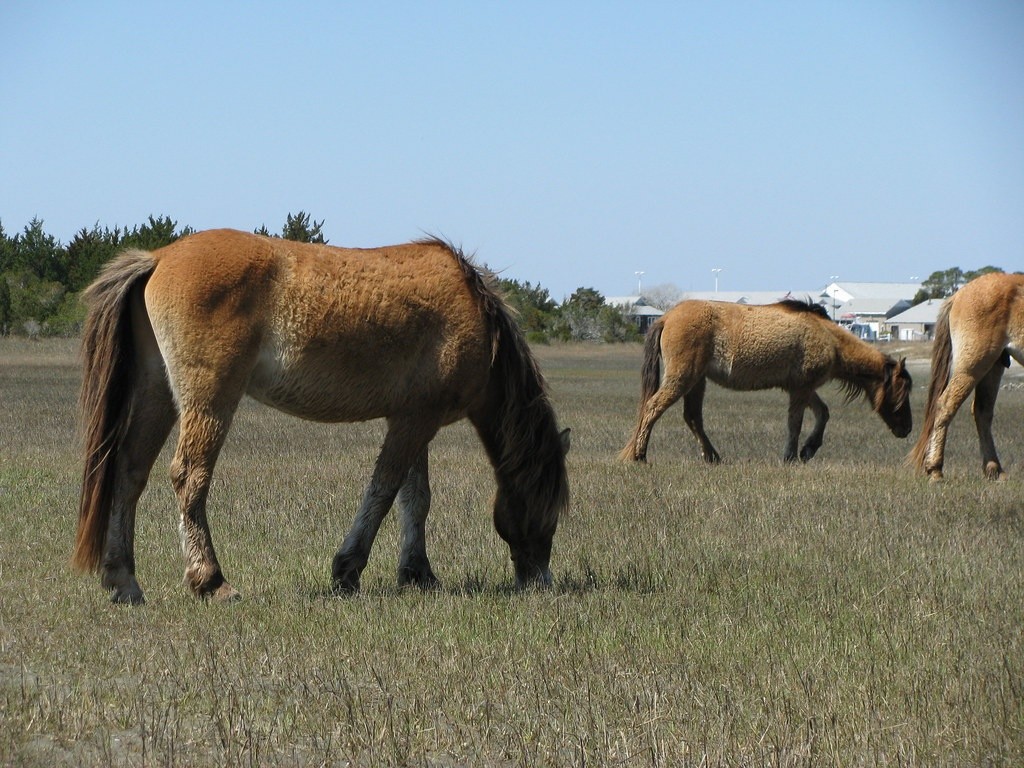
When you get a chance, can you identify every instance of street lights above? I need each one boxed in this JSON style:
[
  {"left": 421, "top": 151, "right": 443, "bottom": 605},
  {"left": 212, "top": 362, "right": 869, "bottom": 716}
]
[
  {"left": 634, "top": 271, "right": 646, "bottom": 297},
  {"left": 711, "top": 268, "right": 723, "bottom": 300},
  {"left": 829, "top": 275, "right": 840, "bottom": 322}
]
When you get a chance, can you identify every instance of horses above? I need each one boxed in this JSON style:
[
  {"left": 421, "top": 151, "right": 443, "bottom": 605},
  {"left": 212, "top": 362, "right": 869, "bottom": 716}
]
[
  {"left": 69, "top": 227, "right": 574, "bottom": 609},
  {"left": 615, "top": 293, "right": 915, "bottom": 468},
  {"left": 899, "top": 272, "right": 1024, "bottom": 488}
]
